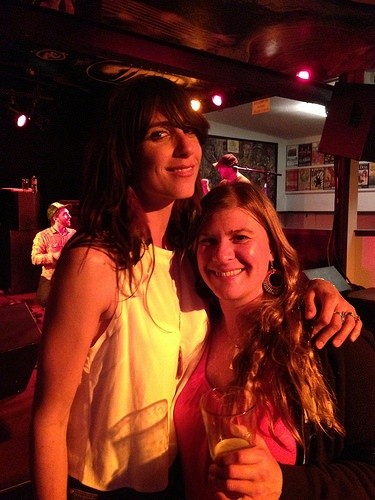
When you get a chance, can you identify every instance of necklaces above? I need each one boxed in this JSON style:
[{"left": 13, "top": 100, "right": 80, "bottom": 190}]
[{"left": 225, "top": 331, "right": 247, "bottom": 348}]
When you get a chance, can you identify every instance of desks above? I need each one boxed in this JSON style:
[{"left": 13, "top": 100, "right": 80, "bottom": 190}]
[{"left": 347, "top": 288, "right": 375, "bottom": 308}]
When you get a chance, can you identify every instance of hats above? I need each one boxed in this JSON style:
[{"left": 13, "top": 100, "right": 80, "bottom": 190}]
[
  {"left": 48, "top": 202, "right": 72, "bottom": 220},
  {"left": 212, "top": 154, "right": 238, "bottom": 168}
]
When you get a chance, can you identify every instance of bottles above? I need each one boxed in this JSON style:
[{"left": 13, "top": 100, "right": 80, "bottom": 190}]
[{"left": 31, "top": 176, "right": 37, "bottom": 192}]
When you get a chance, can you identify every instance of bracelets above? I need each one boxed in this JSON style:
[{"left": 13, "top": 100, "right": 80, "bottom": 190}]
[{"left": 308, "top": 277, "right": 335, "bottom": 287}]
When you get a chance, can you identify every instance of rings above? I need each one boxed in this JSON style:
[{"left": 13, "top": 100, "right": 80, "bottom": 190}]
[
  {"left": 356, "top": 316, "right": 361, "bottom": 321},
  {"left": 345, "top": 311, "right": 355, "bottom": 319},
  {"left": 334, "top": 311, "right": 345, "bottom": 323}
]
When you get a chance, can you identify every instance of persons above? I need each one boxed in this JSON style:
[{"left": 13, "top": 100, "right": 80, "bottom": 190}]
[
  {"left": 29, "top": 76, "right": 362, "bottom": 500},
  {"left": 200, "top": 154, "right": 251, "bottom": 195},
  {"left": 31, "top": 202, "right": 76, "bottom": 307},
  {"left": 172, "top": 182, "right": 375, "bottom": 500}
]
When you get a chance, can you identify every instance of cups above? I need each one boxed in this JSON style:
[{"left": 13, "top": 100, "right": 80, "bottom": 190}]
[
  {"left": 200, "top": 386, "right": 258, "bottom": 462},
  {"left": 22, "top": 179, "right": 29, "bottom": 190}
]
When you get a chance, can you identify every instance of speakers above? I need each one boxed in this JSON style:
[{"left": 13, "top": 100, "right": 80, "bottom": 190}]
[
  {"left": 318, "top": 82, "right": 375, "bottom": 162},
  {"left": 301, "top": 266, "right": 352, "bottom": 296},
  {"left": 0, "top": 302, "right": 42, "bottom": 357}
]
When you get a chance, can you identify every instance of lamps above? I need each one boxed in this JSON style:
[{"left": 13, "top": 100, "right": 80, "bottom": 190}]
[{"left": 6, "top": 101, "right": 28, "bottom": 131}]
